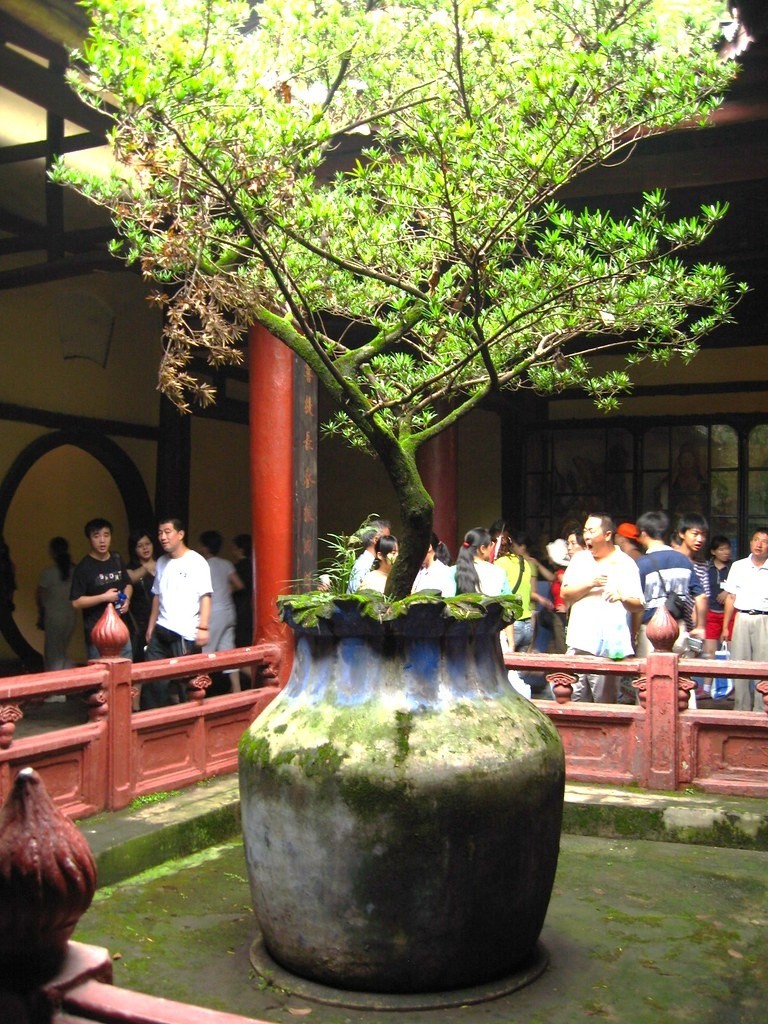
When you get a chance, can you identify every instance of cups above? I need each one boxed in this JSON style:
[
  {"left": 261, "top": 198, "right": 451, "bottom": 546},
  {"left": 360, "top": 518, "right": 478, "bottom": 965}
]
[{"left": 114, "top": 594, "right": 126, "bottom": 616}]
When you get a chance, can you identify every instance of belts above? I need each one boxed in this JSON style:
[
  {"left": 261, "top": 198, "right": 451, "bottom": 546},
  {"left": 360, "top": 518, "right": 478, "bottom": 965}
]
[{"left": 738, "top": 609, "right": 768, "bottom": 616}]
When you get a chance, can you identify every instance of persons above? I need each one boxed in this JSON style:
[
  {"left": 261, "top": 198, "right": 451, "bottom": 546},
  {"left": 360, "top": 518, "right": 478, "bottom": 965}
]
[
  {"left": 200, "top": 531, "right": 243, "bottom": 693},
  {"left": 35, "top": 537, "right": 82, "bottom": 702},
  {"left": 230, "top": 534, "right": 253, "bottom": 692},
  {"left": 411, "top": 531, "right": 452, "bottom": 598},
  {"left": 720, "top": 528, "right": 768, "bottom": 713},
  {"left": 346, "top": 520, "right": 398, "bottom": 593},
  {"left": 488, "top": 518, "right": 553, "bottom": 698},
  {"left": 70, "top": 519, "right": 133, "bottom": 721},
  {"left": 125, "top": 529, "right": 172, "bottom": 712},
  {"left": 450, "top": 527, "right": 514, "bottom": 654},
  {"left": 146, "top": 519, "right": 214, "bottom": 710},
  {"left": 696, "top": 536, "right": 740, "bottom": 701},
  {"left": 546, "top": 511, "right": 711, "bottom": 709}
]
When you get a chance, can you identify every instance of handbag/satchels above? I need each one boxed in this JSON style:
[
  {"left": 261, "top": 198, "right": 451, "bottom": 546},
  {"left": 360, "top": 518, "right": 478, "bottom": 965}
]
[
  {"left": 666, "top": 591, "right": 684, "bottom": 617},
  {"left": 156, "top": 625, "right": 177, "bottom": 645},
  {"left": 708, "top": 640, "right": 734, "bottom": 701},
  {"left": 35, "top": 606, "right": 45, "bottom": 630}
]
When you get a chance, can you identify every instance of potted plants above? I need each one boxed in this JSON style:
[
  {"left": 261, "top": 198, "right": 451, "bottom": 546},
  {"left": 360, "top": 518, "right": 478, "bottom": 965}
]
[{"left": 41, "top": 2, "right": 748, "bottom": 996}]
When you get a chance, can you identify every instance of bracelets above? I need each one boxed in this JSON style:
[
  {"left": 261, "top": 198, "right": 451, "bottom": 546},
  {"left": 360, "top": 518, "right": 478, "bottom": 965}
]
[{"left": 196, "top": 627, "right": 207, "bottom": 631}]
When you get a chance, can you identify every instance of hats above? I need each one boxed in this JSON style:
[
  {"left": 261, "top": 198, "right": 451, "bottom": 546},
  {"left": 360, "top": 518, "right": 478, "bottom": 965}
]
[
  {"left": 616, "top": 523, "right": 642, "bottom": 544},
  {"left": 546, "top": 539, "right": 571, "bottom": 566}
]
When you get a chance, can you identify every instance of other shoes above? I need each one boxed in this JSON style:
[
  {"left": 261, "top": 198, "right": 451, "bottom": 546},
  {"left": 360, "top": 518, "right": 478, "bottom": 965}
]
[
  {"left": 42, "top": 694, "right": 66, "bottom": 703},
  {"left": 697, "top": 689, "right": 710, "bottom": 700},
  {"left": 539, "top": 608, "right": 553, "bottom": 631}
]
